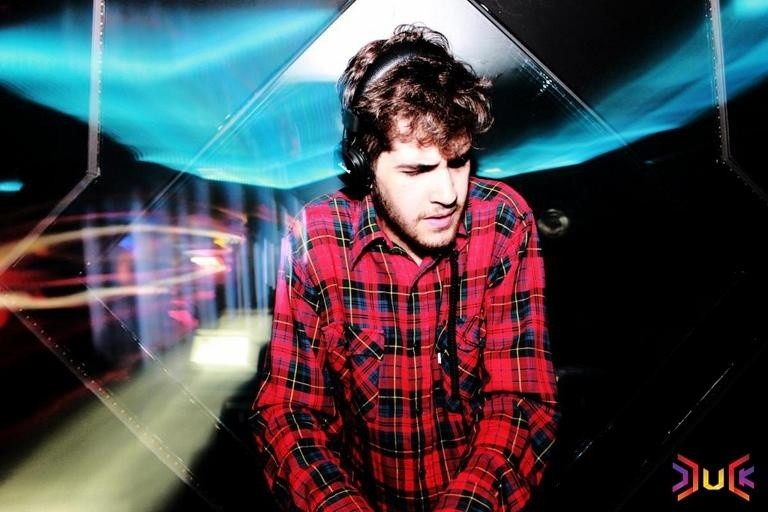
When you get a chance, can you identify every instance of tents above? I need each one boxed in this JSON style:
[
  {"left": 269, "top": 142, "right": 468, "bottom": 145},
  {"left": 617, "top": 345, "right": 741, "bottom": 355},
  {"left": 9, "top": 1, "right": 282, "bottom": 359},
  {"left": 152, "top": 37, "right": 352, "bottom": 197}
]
[{"left": 334, "top": 42, "right": 436, "bottom": 188}]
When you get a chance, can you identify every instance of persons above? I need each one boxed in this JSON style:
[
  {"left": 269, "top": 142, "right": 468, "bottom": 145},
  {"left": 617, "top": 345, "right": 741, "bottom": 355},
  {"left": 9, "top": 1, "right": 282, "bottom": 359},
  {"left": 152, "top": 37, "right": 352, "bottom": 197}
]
[{"left": 246, "top": 21, "right": 562, "bottom": 512}]
